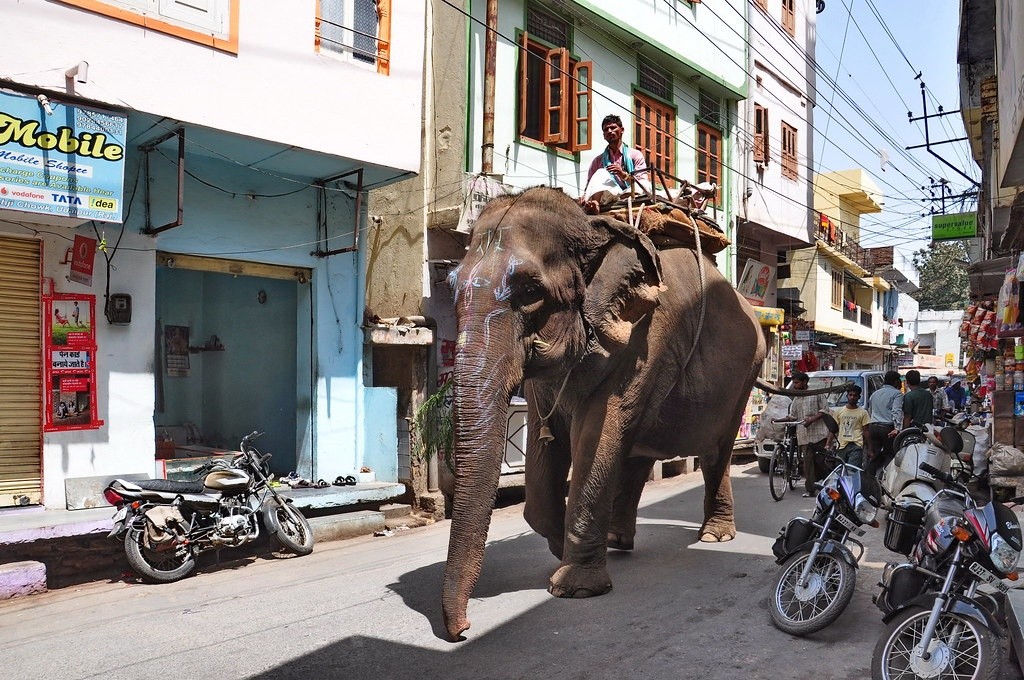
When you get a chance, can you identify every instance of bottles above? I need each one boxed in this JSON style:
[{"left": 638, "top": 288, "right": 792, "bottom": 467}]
[
  {"left": 996, "top": 372, "right": 1003, "bottom": 392},
  {"left": 1013, "top": 370, "right": 1024, "bottom": 390},
  {"left": 1004, "top": 371, "right": 1012, "bottom": 391}
]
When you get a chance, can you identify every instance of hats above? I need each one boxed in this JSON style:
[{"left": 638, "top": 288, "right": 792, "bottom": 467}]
[{"left": 951, "top": 378, "right": 961, "bottom": 387}]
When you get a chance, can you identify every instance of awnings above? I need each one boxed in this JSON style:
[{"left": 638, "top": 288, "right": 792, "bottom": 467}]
[{"left": 967, "top": 254, "right": 1019, "bottom": 302}]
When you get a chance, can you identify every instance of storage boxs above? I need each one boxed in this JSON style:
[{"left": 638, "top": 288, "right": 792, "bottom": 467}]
[{"left": 885, "top": 507, "right": 922, "bottom": 555}]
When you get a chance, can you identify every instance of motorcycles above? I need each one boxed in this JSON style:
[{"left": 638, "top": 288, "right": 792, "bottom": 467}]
[
  {"left": 871, "top": 426, "right": 1023, "bottom": 680},
  {"left": 876, "top": 417, "right": 973, "bottom": 552},
  {"left": 768, "top": 413, "right": 881, "bottom": 636},
  {"left": 103, "top": 431, "right": 314, "bottom": 585}
]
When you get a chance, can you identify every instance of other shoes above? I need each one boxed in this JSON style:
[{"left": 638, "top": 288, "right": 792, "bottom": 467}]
[{"left": 802, "top": 492, "right": 816, "bottom": 497}]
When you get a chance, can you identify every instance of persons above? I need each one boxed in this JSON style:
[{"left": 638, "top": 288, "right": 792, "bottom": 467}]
[
  {"left": 868, "top": 370, "right": 974, "bottom": 482},
  {"left": 583, "top": 116, "right": 649, "bottom": 214},
  {"left": 771, "top": 374, "right": 830, "bottom": 495},
  {"left": 833, "top": 386, "right": 874, "bottom": 467}
]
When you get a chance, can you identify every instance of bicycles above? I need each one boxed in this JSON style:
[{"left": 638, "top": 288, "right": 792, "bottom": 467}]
[{"left": 769, "top": 417, "right": 806, "bottom": 501}]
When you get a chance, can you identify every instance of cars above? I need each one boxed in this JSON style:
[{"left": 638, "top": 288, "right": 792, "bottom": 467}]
[{"left": 753, "top": 369, "right": 886, "bottom": 473}]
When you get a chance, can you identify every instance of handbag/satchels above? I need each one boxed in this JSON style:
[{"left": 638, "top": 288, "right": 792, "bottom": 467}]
[{"left": 144, "top": 506, "right": 184, "bottom": 552}]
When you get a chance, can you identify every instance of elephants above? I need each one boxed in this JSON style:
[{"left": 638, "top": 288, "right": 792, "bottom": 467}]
[{"left": 443, "top": 186, "right": 766, "bottom": 642}]
